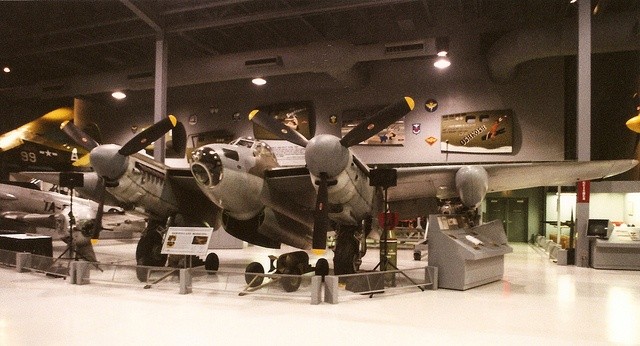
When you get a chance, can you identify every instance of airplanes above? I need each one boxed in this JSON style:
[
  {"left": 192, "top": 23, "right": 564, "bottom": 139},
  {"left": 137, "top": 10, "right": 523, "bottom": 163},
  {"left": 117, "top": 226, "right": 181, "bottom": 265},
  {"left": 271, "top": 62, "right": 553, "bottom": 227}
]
[
  {"left": 0, "top": 138, "right": 151, "bottom": 263},
  {"left": 19, "top": 97, "right": 639, "bottom": 291}
]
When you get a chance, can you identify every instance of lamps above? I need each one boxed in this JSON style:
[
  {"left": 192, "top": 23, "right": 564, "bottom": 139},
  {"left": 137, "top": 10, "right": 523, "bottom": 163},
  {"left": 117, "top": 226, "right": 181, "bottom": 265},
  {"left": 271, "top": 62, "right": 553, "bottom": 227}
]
[
  {"left": 3, "top": 67, "right": 9, "bottom": 72},
  {"left": 111, "top": 92, "right": 126, "bottom": 100},
  {"left": 252, "top": 78, "right": 267, "bottom": 86},
  {"left": 432, "top": 48, "right": 452, "bottom": 70}
]
